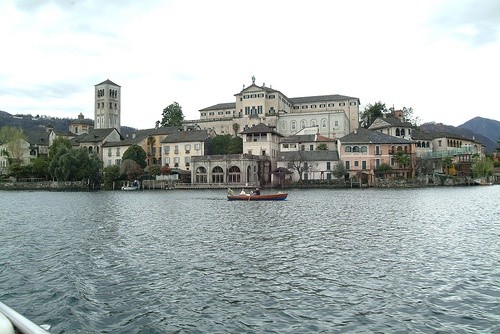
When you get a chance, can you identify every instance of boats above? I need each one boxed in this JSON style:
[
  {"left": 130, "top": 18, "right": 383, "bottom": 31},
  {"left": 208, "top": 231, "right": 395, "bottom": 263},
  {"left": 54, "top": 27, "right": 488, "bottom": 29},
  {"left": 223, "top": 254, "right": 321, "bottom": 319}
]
[
  {"left": 227, "top": 192, "right": 288, "bottom": 200},
  {"left": 472, "top": 177, "right": 495, "bottom": 185},
  {"left": 121, "top": 186, "right": 138, "bottom": 190}
]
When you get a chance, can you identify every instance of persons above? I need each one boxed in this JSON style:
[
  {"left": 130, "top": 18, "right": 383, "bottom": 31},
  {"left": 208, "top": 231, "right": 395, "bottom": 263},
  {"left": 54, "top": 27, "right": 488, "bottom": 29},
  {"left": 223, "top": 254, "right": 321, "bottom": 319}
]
[
  {"left": 240, "top": 188, "right": 246, "bottom": 194},
  {"left": 252, "top": 187, "right": 260, "bottom": 195}
]
